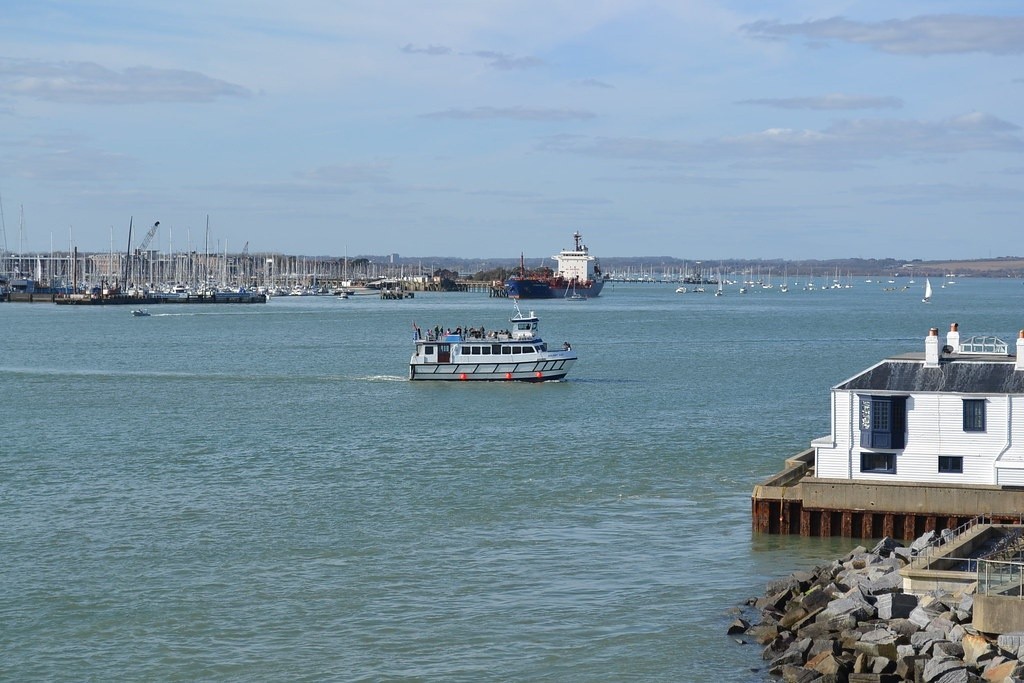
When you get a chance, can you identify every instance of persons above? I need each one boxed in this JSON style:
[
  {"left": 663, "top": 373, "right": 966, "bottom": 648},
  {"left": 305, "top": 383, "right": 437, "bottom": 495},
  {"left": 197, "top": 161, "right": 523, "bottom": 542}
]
[
  {"left": 417, "top": 325, "right": 421, "bottom": 339},
  {"left": 427, "top": 324, "right": 510, "bottom": 340},
  {"left": 526, "top": 324, "right": 530, "bottom": 330}
]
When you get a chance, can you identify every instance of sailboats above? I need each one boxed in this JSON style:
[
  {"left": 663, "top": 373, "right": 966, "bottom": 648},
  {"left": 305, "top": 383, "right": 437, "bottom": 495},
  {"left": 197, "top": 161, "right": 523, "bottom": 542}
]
[
  {"left": 714, "top": 270, "right": 723, "bottom": 296},
  {"left": 794, "top": 268, "right": 799, "bottom": 287},
  {"left": 743, "top": 266, "right": 761, "bottom": 284},
  {"left": 761, "top": 267, "right": 773, "bottom": 288},
  {"left": 921, "top": 277, "right": 933, "bottom": 304},
  {"left": 804, "top": 266, "right": 816, "bottom": 291},
  {"left": 663, "top": 260, "right": 735, "bottom": 284},
  {"left": 606, "top": 264, "right": 656, "bottom": 281},
  {"left": 571, "top": 272, "right": 587, "bottom": 301},
  {"left": 780, "top": 267, "right": 789, "bottom": 293},
  {"left": 0, "top": 199, "right": 355, "bottom": 299},
  {"left": 822, "top": 266, "right": 843, "bottom": 289},
  {"left": 692, "top": 269, "right": 704, "bottom": 292}
]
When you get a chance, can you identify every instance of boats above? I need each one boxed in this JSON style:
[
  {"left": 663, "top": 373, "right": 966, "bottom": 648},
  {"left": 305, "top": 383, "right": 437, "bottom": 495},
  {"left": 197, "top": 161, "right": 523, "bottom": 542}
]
[
  {"left": 503, "top": 230, "right": 607, "bottom": 300},
  {"left": 739, "top": 286, "right": 746, "bottom": 293},
  {"left": 410, "top": 297, "right": 578, "bottom": 382},
  {"left": 131, "top": 309, "right": 149, "bottom": 317},
  {"left": 676, "top": 286, "right": 686, "bottom": 293}
]
